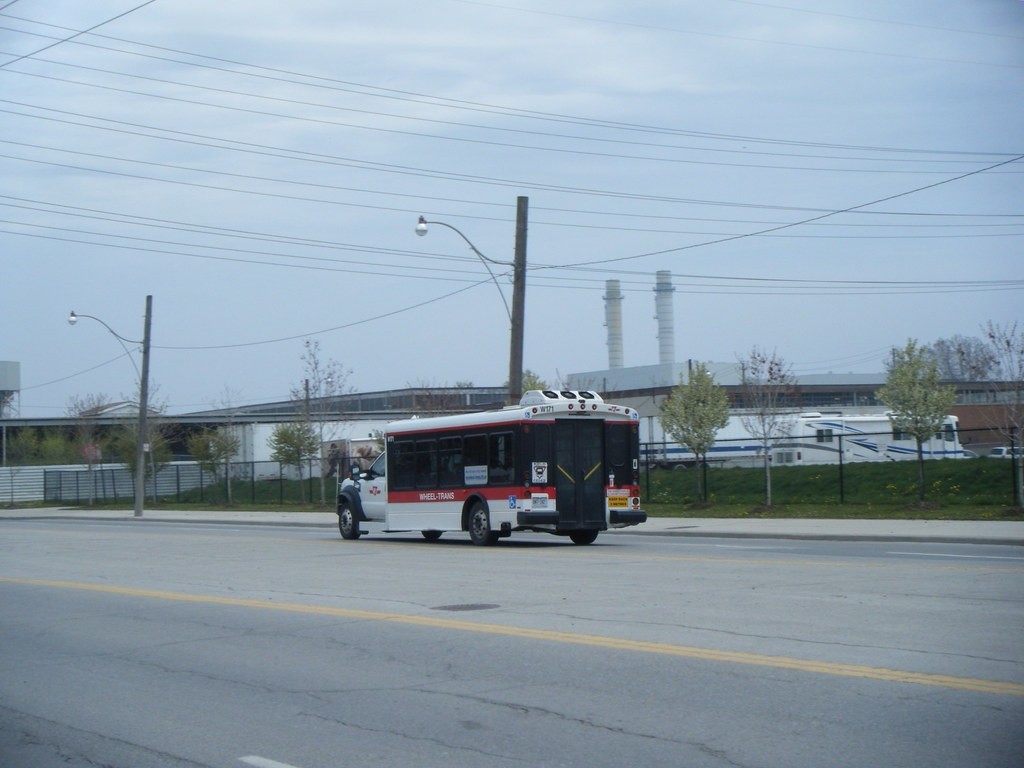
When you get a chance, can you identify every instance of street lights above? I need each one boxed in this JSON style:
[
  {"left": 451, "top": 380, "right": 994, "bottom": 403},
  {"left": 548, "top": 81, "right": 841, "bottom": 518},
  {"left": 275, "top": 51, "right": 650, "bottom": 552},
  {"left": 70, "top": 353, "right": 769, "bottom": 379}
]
[
  {"left": 68, "top": 295, "right": 153, "bottom": 517},
  {"left": 415, "top": 195, "right": 529, "bottom": 406}
]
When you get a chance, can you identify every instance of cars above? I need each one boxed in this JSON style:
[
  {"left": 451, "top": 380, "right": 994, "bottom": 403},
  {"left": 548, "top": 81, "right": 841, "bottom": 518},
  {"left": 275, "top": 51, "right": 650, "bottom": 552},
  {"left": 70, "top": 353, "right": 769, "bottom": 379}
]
[{"left": 988, "top": 447, "right": 1024, "bottom": 461}]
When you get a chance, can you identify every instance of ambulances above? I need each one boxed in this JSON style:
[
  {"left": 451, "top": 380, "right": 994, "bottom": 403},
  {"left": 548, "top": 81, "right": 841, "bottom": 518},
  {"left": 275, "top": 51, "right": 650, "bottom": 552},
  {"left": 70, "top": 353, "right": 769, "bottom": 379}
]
[{"left": 337, "top": 390, "right": 647, "bottom": 545}]
